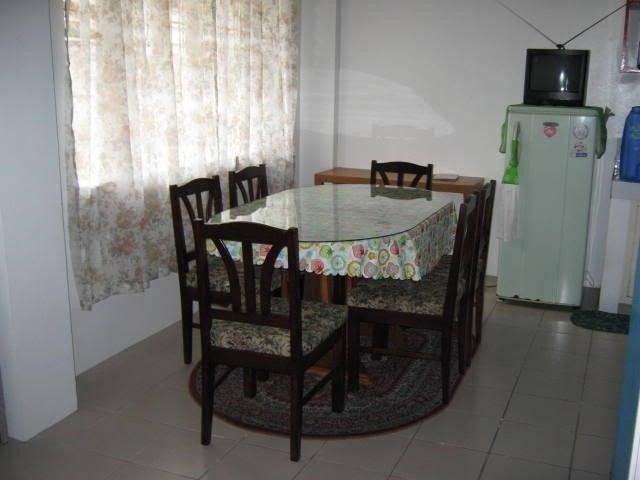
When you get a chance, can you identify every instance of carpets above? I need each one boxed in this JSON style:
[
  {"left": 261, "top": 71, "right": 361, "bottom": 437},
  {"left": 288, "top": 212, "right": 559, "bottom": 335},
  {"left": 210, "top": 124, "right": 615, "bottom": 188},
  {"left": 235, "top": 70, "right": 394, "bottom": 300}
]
[
  {"left": 190, "top": 319, "right": 478, "bottom": 438},
  {"left": 572, "top": 309, "right": 630, "bottom": 334}
]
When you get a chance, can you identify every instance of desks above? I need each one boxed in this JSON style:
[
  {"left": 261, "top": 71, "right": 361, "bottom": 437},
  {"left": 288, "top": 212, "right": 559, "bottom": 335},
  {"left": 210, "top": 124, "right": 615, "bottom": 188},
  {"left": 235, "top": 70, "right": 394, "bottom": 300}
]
[{"left": 314, "top": 167, "right": 484, "bottom": 200}]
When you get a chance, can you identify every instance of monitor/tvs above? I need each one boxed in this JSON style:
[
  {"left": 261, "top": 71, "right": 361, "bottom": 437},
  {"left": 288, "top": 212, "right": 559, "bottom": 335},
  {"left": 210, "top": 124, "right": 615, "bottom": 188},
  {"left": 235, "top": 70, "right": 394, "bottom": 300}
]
[{"left": 522, "top": 48, "right": 590, "bottom": 107}]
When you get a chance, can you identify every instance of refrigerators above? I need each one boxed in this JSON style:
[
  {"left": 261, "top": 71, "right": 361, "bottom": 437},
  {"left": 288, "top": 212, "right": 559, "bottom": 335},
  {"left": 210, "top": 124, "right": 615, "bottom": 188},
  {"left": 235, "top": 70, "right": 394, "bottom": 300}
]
[{"left": 496, "top": 104, "right": 603, "bottom": 312}]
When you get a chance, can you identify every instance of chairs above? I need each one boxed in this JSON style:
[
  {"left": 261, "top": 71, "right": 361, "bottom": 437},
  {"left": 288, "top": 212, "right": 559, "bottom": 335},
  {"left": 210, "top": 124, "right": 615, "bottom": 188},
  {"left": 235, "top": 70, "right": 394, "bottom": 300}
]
[
  {"left": 229, "top": 164, "right": 268, "bottom": 208},
  {"left": 370, "top": 160, "right": 433, "bottom": 191},
  {"left": 430, "top": 179, "right": 496, "bottom": 368},
  {"left": 170, "top": 175, "right": 283, "bottom": 383},
  {"left": 191, "top": 217, "right": 349, "bottom": 462},
  {"left": 344, "top": 189, "right": 481, "bottom": 399}
]
